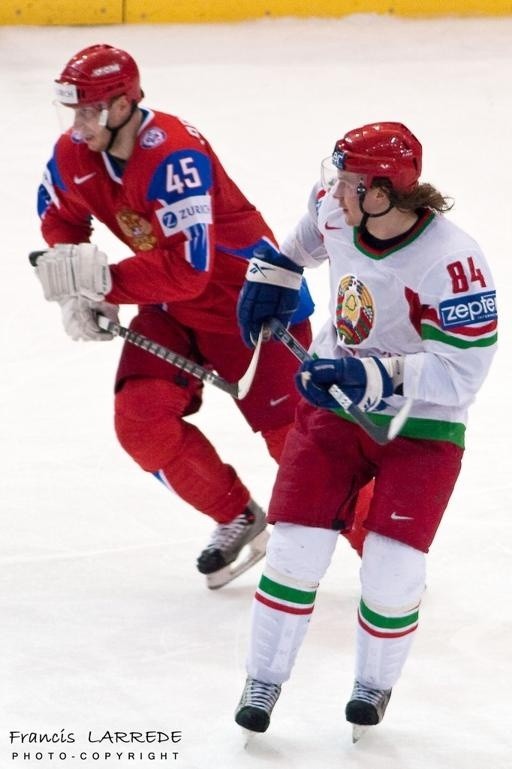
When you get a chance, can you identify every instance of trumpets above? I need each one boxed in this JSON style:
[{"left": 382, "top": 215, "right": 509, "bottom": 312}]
[{"left": 88, "top": 310, "right": 263, "bottom": 401}]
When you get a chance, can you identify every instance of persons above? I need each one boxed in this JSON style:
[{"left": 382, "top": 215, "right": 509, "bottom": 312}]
[
  {"left": 38, "top": 44, "right": 374, "bottom": 575},
  {"left": 235, "top": 122, "right": 498, "bottom": 733}
]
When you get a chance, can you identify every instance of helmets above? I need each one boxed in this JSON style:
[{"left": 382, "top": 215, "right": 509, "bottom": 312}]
[
  {"left": 52, "top": 43, "right": 143, "bottom": 105},
  {"left": 330, "top": 120, "right": 425, "bottom": 191}
]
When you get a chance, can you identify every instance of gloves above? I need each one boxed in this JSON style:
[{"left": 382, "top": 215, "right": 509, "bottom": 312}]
[
  {"left": 294, "top": 352, "right": 394, "bottom": 409},
  {"left": 59, "top": 296, "right": 121, "bottom": 344},
  {"left": 34, "top": 243, "right": 112, "bottom": 301},
  {"left": 236, "top": 237, "right": 304, "bottom": 349}
]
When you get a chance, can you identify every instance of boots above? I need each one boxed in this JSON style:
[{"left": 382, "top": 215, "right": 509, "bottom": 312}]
[
  {"left": 233, "top": 673, "right": 281, "bottom": 732},
  {"left": 343, "top": 681, "right": 392, "bottom": 726},
  {"left": 195, "top": 498, "right": 269, "bottom": 575}
]
